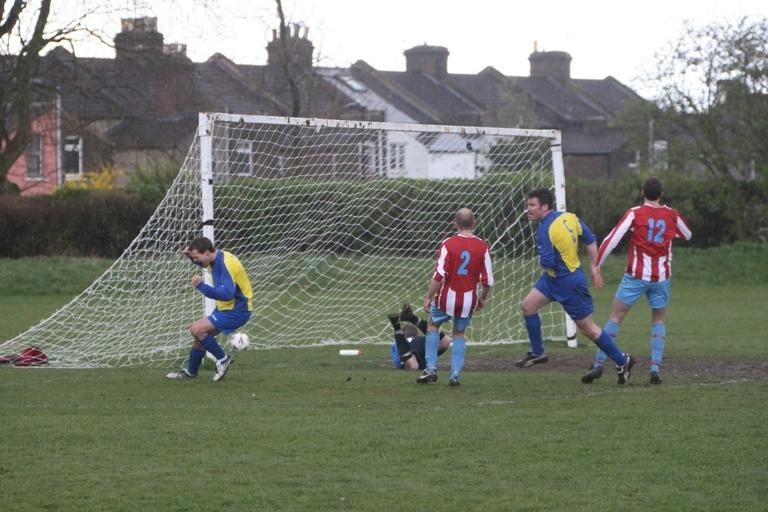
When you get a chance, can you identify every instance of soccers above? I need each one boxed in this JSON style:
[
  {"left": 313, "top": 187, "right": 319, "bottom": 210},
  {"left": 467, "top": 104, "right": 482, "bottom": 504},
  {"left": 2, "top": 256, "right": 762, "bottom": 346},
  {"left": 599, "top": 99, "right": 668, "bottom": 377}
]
[{"left": 229, "top": 332, "right": 250, "bottom": 351}]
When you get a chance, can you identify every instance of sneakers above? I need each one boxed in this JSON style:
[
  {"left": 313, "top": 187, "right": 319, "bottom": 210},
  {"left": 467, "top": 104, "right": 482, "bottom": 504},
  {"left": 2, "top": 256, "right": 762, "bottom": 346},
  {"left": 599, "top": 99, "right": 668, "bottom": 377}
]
[
  {"left": 213, "top": 352, "right": 234, "bottom": 381},
  {"left": 416, "top": 369, "right": 437, "bottom": 383},
  {"left": 650, "top": 371, "right": 663, "bottom": 384},
  {"left": 388, "top": 313, "right": 399, "bottom": 325},
  {"left": 448, "top": 377, "right": 460, "bottom": 386},
  {"left": 400, "top": 303, "right": 412, "bottom": 321},
  {"left": 616, "top": 354, "right": 634, "bottom": 385},
  {"left": 166, "top": 368, "right": 195, "bottom": 379},
  {"left": 514, "top": 352, "right": 548, "bottom": 368},
  {"left": 581, "top": 365, "right": 603, "bottom": 383}
]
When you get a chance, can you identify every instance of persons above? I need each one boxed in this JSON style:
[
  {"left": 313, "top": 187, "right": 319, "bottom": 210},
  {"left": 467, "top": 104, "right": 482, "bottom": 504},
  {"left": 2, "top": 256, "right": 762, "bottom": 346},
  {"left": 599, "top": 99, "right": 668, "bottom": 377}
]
[
  {"left": 165, "top": 236, "right": 253, "bottom": 383},
  {"left": 386, "top": 301, "right": 452, "bottom": 371},
  {"left": 580, "top": 176, "right": 693, "bottom": 384},
  {"left": 511, "top": 187, "right": 636, "bottom": 386},
  {"left": 412, "top": 206, "right": 496, "bottom": 387}
]
